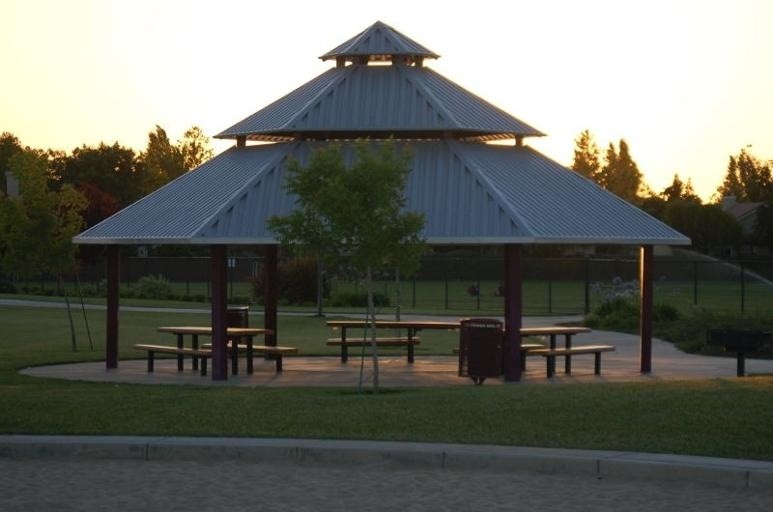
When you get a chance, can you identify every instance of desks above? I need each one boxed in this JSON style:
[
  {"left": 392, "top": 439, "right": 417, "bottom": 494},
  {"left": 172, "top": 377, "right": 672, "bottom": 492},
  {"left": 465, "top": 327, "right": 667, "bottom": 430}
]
[
  {"left": 323, "top": 321, "right": 439, "bottom": 364},
  {"left": 448, "top": 327, "right": 593, "bottom": 375},
  {"left": 157, "top": 327, "right": 271, "bottom": 376}
]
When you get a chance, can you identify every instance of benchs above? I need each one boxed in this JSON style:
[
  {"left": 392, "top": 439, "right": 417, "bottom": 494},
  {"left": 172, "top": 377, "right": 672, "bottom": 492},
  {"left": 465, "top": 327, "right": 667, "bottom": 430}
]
[
  {"left": 326, "top": 337, "right": 421, "bottom": 363},
  {"left": 452, "top": 344, "right": 545, "bottom": 376},
  {"left": 202, "top": 343, "right": 299, "bottom": 374},
  {"left": 527, "top": 344, "right": 616, "bottom": 377},
  {"left": 132, "top": 343, "right": 229, "bottom": 377}
]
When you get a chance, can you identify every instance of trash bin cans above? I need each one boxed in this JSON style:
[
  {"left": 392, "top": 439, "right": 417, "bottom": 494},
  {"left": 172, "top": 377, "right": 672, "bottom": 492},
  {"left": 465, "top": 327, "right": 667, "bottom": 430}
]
[
  {"left": 459, "top": 318, "right": 502, "bottom": 376},
  {"left": 227, "top": 307, "right": 249, "bottom": 340}
]
